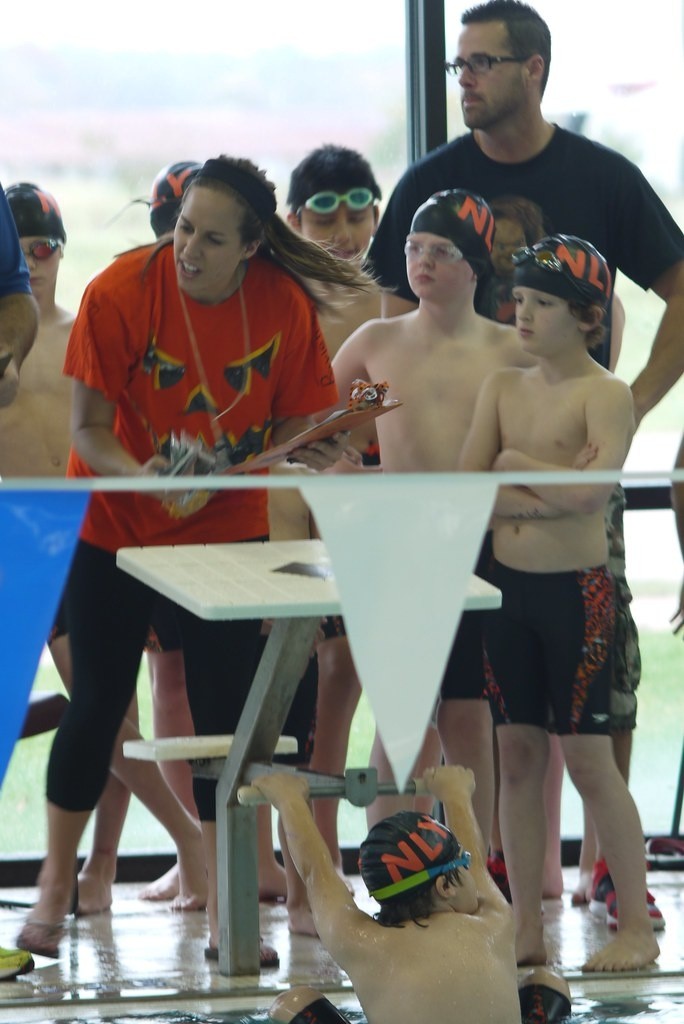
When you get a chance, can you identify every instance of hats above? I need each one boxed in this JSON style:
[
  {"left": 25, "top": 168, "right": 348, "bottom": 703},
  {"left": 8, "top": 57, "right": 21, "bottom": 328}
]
[
  {"left": 411, "top": 188, "right": 496, "bottom": 279},
  {"left": 514, "top": 233, "right": 610, "bottom": 308},
  {"left": 5, "top": 184, "right": 65, "bottom": 242},
  {"left": 150, "top": 161, "right": 204, "bottom": 236},
  {"left": 357, "top": 810, "right": 459, "bottom": 899}
]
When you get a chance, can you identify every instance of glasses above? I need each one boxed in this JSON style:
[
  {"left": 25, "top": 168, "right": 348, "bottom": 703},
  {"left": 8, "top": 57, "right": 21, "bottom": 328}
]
[
  {"left": 305, "top": 188, "right": 374, "bottom": 214},
  {"left": 445, "top": 55, "right": 525, "bottom": 75},
  {"left": 24, "top": 239, "right": 58, "bottom": 260},
  {"left": 460, "top": 848, "right": 470, "bottom": 870},
  {"left": 404, "top": 242, "right": 464, "bottom": 262},
  {"left": 511, "top": 245, "right": 584, "bottom": 292}
]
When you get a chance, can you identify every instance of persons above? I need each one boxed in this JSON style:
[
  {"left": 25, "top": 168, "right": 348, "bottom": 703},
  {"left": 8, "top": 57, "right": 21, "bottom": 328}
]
[
  {"left": 253, "top": 764, "right": 572, "bottom": 1024},
  {"left": 0, "top": 0, "right": 684, "bottom": 975},
  {"left": 458, "top": 232, "right": 661, "bottom": 973}
]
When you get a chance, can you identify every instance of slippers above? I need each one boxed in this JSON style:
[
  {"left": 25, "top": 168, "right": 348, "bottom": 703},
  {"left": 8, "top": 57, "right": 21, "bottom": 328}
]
[
  {"left": 204, "top": 936, "right": 279, "bottom": 967},
  {"left": 16, "top": 917, "right": 67, "bottom": 958}
]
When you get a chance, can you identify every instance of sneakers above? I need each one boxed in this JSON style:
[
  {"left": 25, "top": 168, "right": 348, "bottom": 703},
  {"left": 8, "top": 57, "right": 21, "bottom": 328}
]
[{"left": 589, "top": 858, "right": 666, "bottom": 929}]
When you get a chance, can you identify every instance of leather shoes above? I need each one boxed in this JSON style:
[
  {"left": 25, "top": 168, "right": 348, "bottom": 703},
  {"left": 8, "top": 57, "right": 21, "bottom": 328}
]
[{"left": 0, "top": 947, "right": 35, "bottom": 979}]
